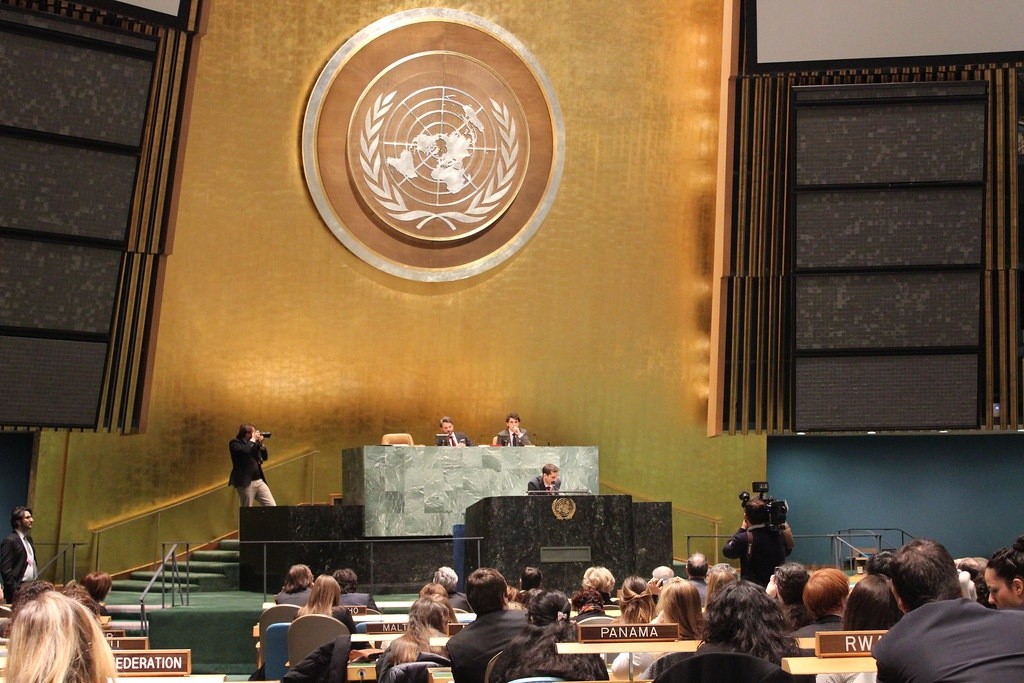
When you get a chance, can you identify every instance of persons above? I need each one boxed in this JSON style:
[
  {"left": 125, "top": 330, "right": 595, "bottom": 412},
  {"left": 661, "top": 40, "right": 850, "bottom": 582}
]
[
  {"left": 528, "top": 464, "right": 561, "bottom": 496},
  {"left": 611, "top": 549, "right": 738, "bottom": 680},
  {"left": 0, "top": 506, "right": 38, "bottom": 604},
  {"left": 446, "top": 568, "right": 527, "bottom": 683},
  {"left": 570, "top": 567, "right": 656, "bottom": 641},
  {"left": 333, "top": 569, "right": 378, "bottom": 612},
  {"left": 4, "top": 590, "right": 117, "bottom": 683},
  {"left": 376, "top": 594, "right": 459, "bottom": 683},
  {"left": 293, "top": 574, "right": 372, "bottom": 649},
  {"left": 228, "top": 422, "right": 276, "bottom": 507},
  {"left": 432, "top": 566, "right": 473, "bottom": 614},
  {"left": 872, "top": 539, "right": 1024, "bottom": 683},
  {"left": 702, "top": 579, "right": 817, "bottom": 683},
  {"left": 419, "top": 582, "right": 449, "bottom": 599},
  {"left": 490, "top": 589, "right": 609, "bottom": 683},
  {"left": 437, "top": 417, "right": 475, "bottom": 447},
  {"left": 766, "top": 551, "right": 904, "bottom": 683},
  {"left": 722, "top": 499, "right": 791, "bottom": 589},
  {"left": 0, "top": 572, "right": 111, "bottom": 640},
  {"left": 275, "top": 564, "right": 315, "bottom": 608},
  {"left": 506, "top": 567, "right": 544, "bottom": 611},
  {"left": 496, "top": 412, "right": 531, "bottom": 447},
  {"left": 954, "top": 535, "right": 1024, "bottom": 612}
]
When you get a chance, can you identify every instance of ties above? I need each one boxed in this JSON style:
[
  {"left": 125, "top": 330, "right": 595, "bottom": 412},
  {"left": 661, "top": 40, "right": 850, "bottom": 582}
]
[
  {"left": 449, "top": 435, "right": 456, "bottom": 446},
  {"left": 23, "top": 537, "right": 36, "bottom": 578},
  {"left": 512, "top": 433, "right": 517, "bottom": 447}
]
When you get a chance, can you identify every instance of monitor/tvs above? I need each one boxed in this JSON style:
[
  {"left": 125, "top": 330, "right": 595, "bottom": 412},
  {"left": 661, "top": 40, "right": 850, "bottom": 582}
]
[
  {"left": 435, "top": 434, "right": 449, "bottom": 446},
  {"left": 498, "top": 434, "right": 510, "bottom": 446}
]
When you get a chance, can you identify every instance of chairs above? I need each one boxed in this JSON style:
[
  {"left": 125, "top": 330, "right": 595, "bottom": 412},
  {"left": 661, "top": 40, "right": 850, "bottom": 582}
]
[
  {"left": 382, "top": 434, "right": 415, "bottom": 446},
  {"left": 259, "top": 604, "right": 802, "bottom": 683}
]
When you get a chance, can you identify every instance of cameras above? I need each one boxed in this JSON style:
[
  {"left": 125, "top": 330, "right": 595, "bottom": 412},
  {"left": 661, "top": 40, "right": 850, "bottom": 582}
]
[{"left": 258, "top": 431, "right": 272, "bottom": 439}]
